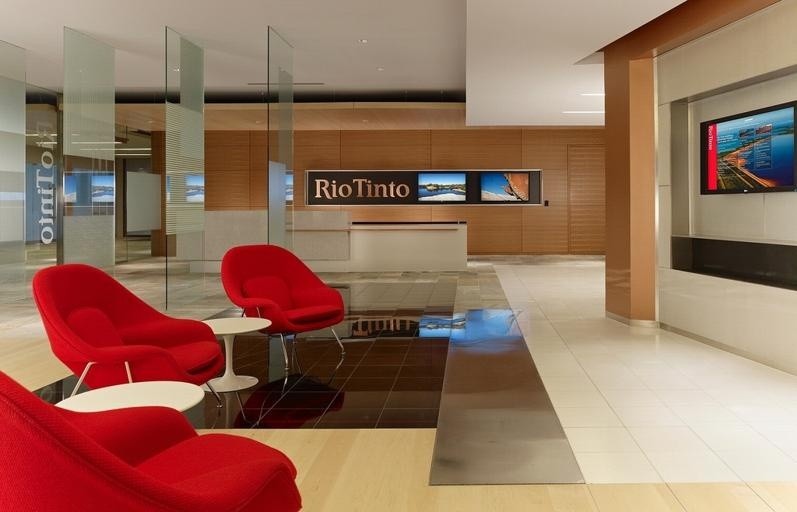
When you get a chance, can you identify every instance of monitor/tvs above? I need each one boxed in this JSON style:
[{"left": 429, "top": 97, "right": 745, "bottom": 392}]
[{"left": 700, "top": 100, "right": 797, "bottom": 195}]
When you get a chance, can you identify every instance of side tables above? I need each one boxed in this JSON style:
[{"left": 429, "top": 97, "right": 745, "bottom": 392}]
[
  {"left": 202, "top": 317, "right": 272, "bottom": 394},
  {"left": 53, "top": 381, "right": 205, "bottom": 413}
]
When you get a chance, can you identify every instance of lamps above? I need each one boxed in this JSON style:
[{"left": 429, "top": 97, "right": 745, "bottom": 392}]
[{"left": 26, "top": 125, "right": 129, "bottom": 145}]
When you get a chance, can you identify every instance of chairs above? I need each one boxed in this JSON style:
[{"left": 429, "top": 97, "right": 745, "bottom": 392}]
[
  {"left": 221, "top": 245, "right": 347, "bottom": 390},
  {"left": 32, "top": 264, "right": 225, "bottom": 409},
  {"left": 1, "top": 370, "right": 302, "bottom": 512}
]
